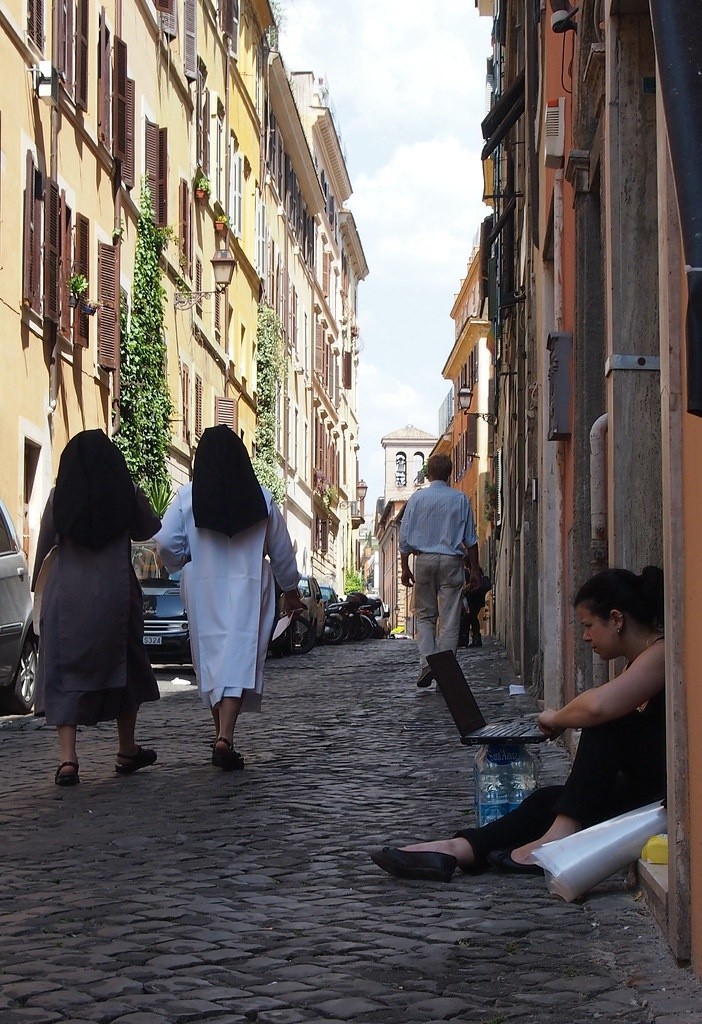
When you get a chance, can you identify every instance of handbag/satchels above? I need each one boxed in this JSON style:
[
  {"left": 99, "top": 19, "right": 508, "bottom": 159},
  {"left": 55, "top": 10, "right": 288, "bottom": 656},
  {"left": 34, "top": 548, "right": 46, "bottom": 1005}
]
[{"left": 480, "top": 576, "right": 492, "bottom": 592}]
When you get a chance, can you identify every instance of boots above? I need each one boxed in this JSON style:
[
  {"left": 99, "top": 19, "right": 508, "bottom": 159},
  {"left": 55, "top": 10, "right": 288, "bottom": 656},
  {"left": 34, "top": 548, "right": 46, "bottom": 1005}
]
[{"left": 469, "top": 633, "right": 482, "bottom": 647}]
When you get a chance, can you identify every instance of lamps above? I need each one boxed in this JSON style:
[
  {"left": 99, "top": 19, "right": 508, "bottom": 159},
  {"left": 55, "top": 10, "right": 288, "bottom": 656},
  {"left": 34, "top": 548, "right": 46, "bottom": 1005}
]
[
  {"left": 340, "top": 478, "right": 368, "bottom": 510},
  {"left": 457, "top": 384, "right": 499, "bottom": 427},
  {"left": 174, "top": 246, "right": 238, "bottom": 311}
]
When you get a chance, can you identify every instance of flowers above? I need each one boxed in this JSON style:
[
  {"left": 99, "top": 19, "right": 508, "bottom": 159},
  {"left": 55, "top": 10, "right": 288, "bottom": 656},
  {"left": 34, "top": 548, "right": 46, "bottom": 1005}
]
[{"left": 215, "top": 215, "right": 228, "bottom": 223}]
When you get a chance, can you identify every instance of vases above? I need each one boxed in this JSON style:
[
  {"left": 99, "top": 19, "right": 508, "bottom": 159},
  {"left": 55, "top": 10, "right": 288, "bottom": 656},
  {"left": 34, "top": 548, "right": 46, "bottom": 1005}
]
[{"left": 216, "top": 222, "right": 224, "bottom": 231}]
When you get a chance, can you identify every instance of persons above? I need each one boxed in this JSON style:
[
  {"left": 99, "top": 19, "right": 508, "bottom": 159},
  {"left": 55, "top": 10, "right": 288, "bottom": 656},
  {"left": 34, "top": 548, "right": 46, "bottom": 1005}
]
[
  {"left": 159, "top": 424, "right": 308, "bottom": 769},
  {"left": 459, "top": 555, "right": 484, "bottom": 648},
  {"left": 31, "top": 429, "right": 162, "bottom": 784},
  {"left": 399, "top": 453, "right": 482, "bottom": 698},
  {"left": 369, "top": 565, "right": 666, "bottom": 883}
]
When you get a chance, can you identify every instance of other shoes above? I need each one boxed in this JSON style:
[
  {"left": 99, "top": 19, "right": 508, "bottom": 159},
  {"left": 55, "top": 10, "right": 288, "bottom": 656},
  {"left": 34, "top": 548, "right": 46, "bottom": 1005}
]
[
  {"left": 417, "top": 665, "right": 434, "bottom": 687},
  {"left": 370, "top": 847, "right": 457, "bottom": 882},
  {"left": 487, "top": 846, "right": 544, "bottom": 875}
]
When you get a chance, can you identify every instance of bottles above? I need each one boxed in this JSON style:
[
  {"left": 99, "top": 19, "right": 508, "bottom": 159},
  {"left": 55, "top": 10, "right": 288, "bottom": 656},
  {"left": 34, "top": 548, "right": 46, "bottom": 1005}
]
[
  {"left": 474, "top": 743, "right": 510, "bottom": 829},
  {"left": 510, "top": 744, "right": 537, "bottom": 814}
]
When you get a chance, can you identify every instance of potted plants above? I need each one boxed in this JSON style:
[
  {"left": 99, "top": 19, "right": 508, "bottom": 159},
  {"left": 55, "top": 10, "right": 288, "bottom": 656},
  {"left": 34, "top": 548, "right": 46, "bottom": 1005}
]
[
  {"left": 66, "top": 273, "right": 89, "bottom": 308},
  {"left": 83, "top": 299, "right": 102, "bottom": 315},
  {"left": 483, "top": 480, "right": 496, "bottom": 499},
  {"left": 196, "top": 177, "right": 211, "bottom": 206}
]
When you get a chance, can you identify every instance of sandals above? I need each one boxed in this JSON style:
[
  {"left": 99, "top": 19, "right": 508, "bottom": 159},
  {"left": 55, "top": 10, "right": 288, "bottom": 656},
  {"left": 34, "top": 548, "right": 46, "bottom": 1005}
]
[
  {"left": 115, "top": 745, "right": 157, "bottom": 773},
  {"left": 212, "top": 737, "right": 245, "bottom": 771},
  {"left": 54, "top": 762, "right": 80, "bottom": 786}
]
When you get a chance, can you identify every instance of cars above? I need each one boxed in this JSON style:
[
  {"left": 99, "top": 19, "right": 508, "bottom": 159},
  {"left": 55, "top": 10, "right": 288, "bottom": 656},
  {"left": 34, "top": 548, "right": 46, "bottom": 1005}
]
[
  {"left": 131, "top": 540, "right": 196, "bottom": 665},
  {"left": 0, "top": 502, "right": 39, "bottom": 715},
  {"left": 281, "top": 573, "right": 391, "bottom": 656}
]
